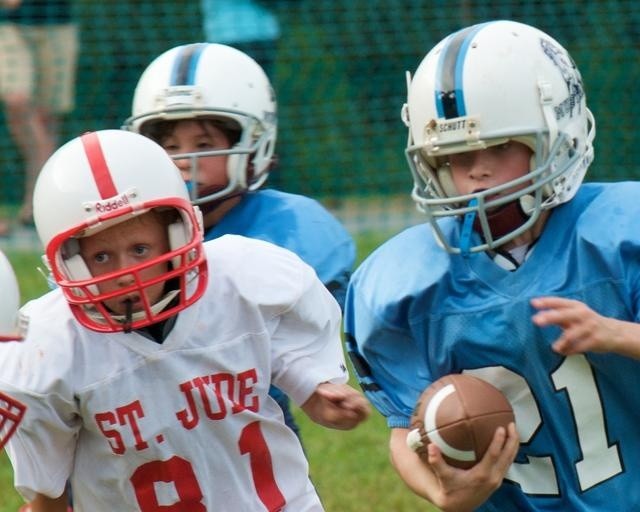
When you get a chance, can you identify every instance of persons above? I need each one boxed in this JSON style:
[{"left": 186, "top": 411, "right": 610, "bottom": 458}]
[
  {"left": 199, "top": 0, "right": 281, "bottom": 80},
  {"left": 344, "top": 20, "right": 640, "bottom": 512},
  {"left": 0, "top": 0, "right": 78, "bottom": 225},
  {"left": 122, "top": 43, "right": 359, "bottom": 456},
  {"left": 0, "top": 129, "right": 369, "bottom": 512}
]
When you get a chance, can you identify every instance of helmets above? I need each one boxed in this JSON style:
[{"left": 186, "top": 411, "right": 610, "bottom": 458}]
[
  {"left": 32, "top": 129, "right": 209, "bottom": 333},
  {"left": 119, "top": 42, "right": 278, "bottom": 206},
  {"left": 400, "top": 19, "right": 597, "bottom": 259}
]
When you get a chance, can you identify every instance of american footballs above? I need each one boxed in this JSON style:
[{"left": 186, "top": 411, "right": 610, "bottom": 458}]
[{"left": 407, "top": 373, "right": 515, "bottom": 470}]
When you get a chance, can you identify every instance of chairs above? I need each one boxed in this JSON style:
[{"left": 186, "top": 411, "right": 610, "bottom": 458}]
[{"left": 0, "top": 0, "right": 640, "bottom": 221}]
